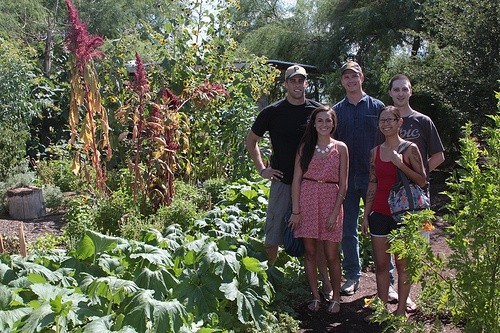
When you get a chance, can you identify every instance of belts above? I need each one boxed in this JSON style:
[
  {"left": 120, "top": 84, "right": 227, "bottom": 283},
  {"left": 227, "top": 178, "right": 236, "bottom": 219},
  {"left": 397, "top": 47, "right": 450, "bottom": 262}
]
[{"left": 303, "top": 178, "right": 339, "bottom": 184}]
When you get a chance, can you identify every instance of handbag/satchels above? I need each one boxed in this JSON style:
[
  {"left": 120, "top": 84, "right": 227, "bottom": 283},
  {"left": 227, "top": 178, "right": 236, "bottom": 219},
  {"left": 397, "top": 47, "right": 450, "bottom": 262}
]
[
  {"left": 282, "top": 217, "right": 304, "bottom": 257},
  {"left": 388, "top": 141, "right": 431, "bottom": 221}
]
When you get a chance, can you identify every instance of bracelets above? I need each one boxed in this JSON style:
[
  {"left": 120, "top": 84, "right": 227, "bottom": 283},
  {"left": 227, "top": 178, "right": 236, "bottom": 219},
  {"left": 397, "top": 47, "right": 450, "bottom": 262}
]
[
  {"left": 260, "top": 168, "right": 266, "bottom": 175},
  {"left": 292, "top": 212, "right": 300, "bottom": 215},
  {"left": 338, "top": 193, "right": 345, "bottom": 200}
]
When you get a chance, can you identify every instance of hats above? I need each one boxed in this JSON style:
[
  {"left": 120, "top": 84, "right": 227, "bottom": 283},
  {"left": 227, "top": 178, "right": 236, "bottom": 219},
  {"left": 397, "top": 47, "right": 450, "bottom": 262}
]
[
  {"left": 340, "top": 61, "right": 362, "bottom": 74},
  {"left": 285, "top": 65, "right": 307, "bottom": 80}
]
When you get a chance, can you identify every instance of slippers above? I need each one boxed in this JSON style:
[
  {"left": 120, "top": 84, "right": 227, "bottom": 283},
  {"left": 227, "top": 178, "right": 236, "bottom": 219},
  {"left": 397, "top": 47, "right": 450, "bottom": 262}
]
[
  {"left": 327, "top": 300, "right": 340, "bottom": 314},
  {"left": 308, "top": 299, "right": 320, "bottom": 312},
  {"left": 404, "top": 296, "right": 417, "bottom": 313}
]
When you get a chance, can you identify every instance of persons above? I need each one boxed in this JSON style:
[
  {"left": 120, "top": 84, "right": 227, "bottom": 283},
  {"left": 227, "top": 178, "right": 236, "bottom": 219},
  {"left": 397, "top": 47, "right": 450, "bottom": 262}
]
[
  {"left": 245, "top": 65, "right": 332, "bottom": 300},
  {"left": 287, "top": 106, "right": 349, "bottom": 313},
  {"left": 330, "top": 62, "right": 397, "bottom": 303},
  {"left": 387, "top": 74, "right": 446, "bottom": 312},
  {"left": 363, "top": 106, "right": 426, "bottom": 321}
]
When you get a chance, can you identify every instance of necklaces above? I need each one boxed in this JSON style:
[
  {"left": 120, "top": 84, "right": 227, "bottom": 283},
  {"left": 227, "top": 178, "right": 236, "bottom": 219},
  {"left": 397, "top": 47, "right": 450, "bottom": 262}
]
[{"left": 315, "top": 143, "right": 333, "bottom": 154}]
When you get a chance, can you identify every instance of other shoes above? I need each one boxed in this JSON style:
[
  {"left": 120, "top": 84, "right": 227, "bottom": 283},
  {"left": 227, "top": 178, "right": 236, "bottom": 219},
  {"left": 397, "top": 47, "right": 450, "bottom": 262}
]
[
  {"left": 387, "top": 282, "right": 398, "bottom": 302},
  {"left": 319, "top": 287, "right": 334, "bottom": 302},
  {"left": 339, "top": 278, "right": 360, "bottom": 295}
]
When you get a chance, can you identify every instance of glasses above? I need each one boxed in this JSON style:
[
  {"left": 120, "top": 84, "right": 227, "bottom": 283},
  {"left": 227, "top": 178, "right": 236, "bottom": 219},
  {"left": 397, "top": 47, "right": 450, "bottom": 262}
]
[{"left": 379, "top": 118, "right": 399, "bottom": 123}]
